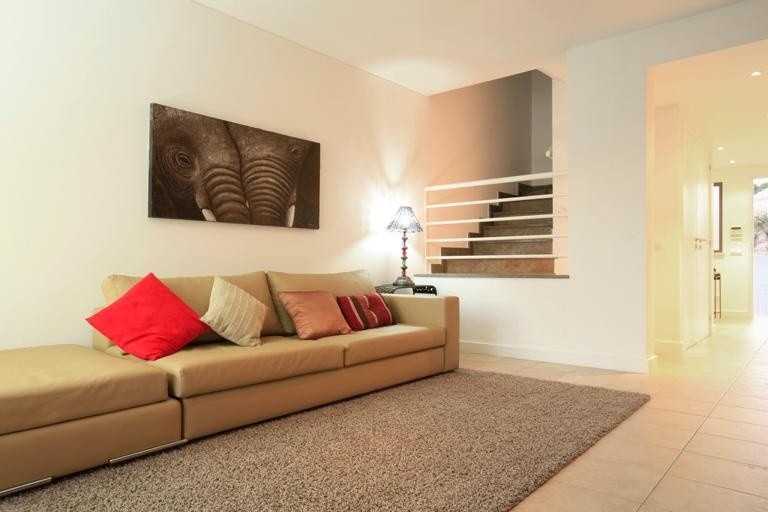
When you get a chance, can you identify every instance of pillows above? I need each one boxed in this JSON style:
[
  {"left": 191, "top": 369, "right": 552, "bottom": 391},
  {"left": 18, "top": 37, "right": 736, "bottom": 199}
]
[
  {"left": 335, "top": 292, "right": 393, "bottom": 332},
  {"left": 85, "top": 273, "right": 212, "bottom": 361},
  {"left": 199, "top": 275, "right": 269, "bottom": 348},
  {"left": 277, "top": 291, "right": 352, "bottom": 340}
]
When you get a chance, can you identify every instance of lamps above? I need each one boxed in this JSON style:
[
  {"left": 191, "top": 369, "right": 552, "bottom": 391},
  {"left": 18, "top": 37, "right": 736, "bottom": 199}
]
[{"left": 383, "top": 205, "right": 425, "bottom": 286}]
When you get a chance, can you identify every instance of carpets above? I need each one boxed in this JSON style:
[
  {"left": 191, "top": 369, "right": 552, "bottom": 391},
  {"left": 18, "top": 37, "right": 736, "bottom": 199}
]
[{"left": 0, "top": 366, "right": 650, "bottom": 512}]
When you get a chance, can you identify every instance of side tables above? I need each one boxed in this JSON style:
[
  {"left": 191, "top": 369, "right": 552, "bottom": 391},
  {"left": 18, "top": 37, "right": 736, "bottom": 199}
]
[{"left": 376, "top": 281, "right": 437, "bottom": 297}]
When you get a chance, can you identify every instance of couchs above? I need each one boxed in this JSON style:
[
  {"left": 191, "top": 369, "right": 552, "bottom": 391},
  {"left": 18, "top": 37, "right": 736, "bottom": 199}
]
[{"left": 95, "top": 270, "right": 459, "bottom": 449}]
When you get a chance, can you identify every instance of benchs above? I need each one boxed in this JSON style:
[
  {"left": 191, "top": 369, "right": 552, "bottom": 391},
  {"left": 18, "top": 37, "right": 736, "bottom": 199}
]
[{"left": 2, "top": 345, "right": 182, "bottom": 496}]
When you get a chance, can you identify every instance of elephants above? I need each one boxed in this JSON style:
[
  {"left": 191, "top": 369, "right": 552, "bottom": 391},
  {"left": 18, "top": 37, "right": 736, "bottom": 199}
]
[{"left": 149, "top": 103, "right": 313, "bottom": 228}]
